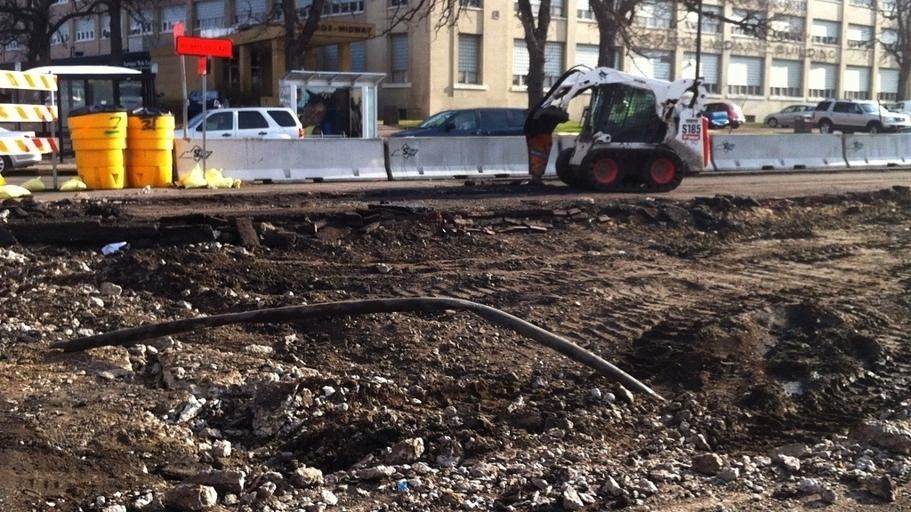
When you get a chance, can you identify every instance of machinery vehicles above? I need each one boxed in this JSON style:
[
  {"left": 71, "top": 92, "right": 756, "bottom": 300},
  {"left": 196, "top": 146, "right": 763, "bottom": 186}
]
[{"left": 520, "top": 60, "right": 714, "bottom": 196}]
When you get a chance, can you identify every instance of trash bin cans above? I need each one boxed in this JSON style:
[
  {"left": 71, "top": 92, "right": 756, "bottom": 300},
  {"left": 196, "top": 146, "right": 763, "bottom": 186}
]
[{"left": 794, "top": 114, "right": 812, "bottom": 133}]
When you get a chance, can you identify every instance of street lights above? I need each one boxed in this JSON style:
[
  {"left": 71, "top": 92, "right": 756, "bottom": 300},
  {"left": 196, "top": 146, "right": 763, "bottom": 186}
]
[{"left": 67, "top": 44, "right": 85, "bottom": 111}]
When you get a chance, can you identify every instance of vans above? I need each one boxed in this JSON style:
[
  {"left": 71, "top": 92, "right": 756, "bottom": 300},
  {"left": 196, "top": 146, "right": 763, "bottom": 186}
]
[{"left": 389, "top": 105, "right": 529, "bottom": 137}]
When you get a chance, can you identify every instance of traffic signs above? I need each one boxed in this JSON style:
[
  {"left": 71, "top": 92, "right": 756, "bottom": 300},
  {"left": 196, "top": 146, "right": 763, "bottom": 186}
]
[{"left": 174, "top": 35, "right": 233, "bottom": 59}]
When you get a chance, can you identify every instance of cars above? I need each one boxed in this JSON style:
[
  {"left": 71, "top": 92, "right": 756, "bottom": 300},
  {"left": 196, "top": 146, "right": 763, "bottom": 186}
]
[{"left": 699, "top": 96, "right": 910, "bottom": 133}]
